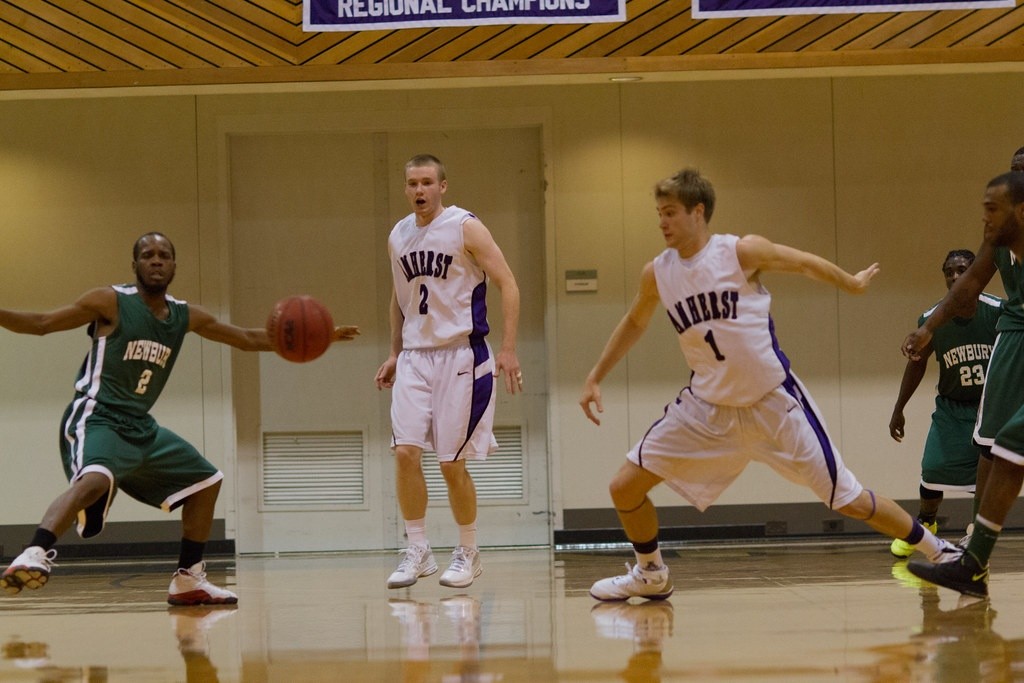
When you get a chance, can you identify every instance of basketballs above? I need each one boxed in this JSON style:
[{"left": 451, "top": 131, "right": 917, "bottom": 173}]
[{"left": 268, "top": 296, "right": 335, "bottom": 365}]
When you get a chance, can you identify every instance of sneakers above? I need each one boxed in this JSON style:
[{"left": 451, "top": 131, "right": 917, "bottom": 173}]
[
  {"left": 590, "top": 603, "right": 673, "bottom": 637},
  {"left": 590, "top": 561, "right": 674, "bottom": 602},
  {"left": 387, "top": 543, "right": 438, "bottom": 589},
  {"left": 928, "top": 538, "right": 964, "bottom": 564},
  {"left": 167, "top": 606, "right": 239, "bottom": 634},
  {"left": 907, "top": 548, "right": 989, "bottom": 598},
  {"left": 890, "top": 519, "right": 938, "bottom": 559},
  {"left": 167, "top": 560, "right": 238, "bottom": 605},
  {"left": 0, "top": 546, "right": 58, "bottom": 596},
  {"left": 439, "top": 544, "right": 482, "bottom": 588}
]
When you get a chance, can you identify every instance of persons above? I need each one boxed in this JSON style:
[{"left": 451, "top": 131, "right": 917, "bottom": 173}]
[
  {"left": 900, "top": 145, "right": 1024, "bottom": 598},
  {"left": 373, "top": 154, "right": 524, "bottom": 589},
  {"left": 887, "top": 247, "right": 1010, "bottom": 558},
  {"left": 578, "top": 167, "right": 965, "bottom": 602},
  {"left": 0, "top": 232, "right": 362, "bottom": 606},
  {"left": 906, "top": 170, "right": 1024, "bottom": 598}
]
